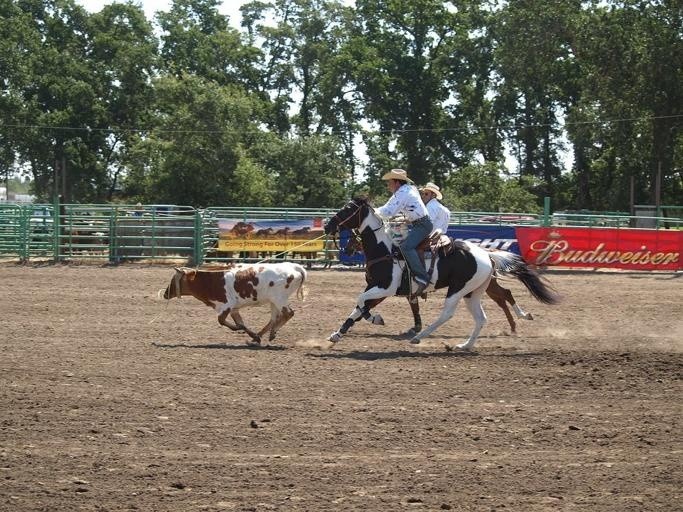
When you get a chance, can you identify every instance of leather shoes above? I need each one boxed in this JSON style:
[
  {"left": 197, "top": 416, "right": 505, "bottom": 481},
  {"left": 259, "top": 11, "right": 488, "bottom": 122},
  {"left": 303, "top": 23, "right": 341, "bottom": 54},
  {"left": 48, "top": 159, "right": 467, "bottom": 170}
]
[{"left": 411, "top": 272, "right": 430, "bottom": 296}]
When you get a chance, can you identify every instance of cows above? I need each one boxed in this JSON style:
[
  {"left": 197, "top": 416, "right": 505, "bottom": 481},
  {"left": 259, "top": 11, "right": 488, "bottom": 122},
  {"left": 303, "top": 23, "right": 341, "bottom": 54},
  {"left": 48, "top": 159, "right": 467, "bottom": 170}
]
[{"left": 163, "top": 262, "right": 307, "bottom": 344}]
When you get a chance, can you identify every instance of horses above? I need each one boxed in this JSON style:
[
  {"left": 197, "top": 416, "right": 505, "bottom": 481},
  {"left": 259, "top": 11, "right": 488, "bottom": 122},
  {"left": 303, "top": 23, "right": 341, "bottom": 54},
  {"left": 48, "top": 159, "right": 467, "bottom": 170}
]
[
  {"left": 293, "top": 227, "right": 311, "bottom": 234},
  {"left": 255, "top": 228, "right": 273, "bottom": 238},
  {"left": 323, "top": 195, "right": 562, "bottom": 352},
  {"left": 276, "top": 226, "right": 290, "bottom": 239}
]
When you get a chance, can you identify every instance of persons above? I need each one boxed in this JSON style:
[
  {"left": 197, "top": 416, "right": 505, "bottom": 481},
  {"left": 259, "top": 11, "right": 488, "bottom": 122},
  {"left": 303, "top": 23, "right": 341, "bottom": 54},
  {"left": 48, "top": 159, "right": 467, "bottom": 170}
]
[
  {"left": 131, "top": 203, "right": 144, "bottom": 260},
  {"left": 421, "top": 182, "right": 451, "bottom": 237},
  {"left": 372, "top": 169, "right": 432, "bottom": 284}
]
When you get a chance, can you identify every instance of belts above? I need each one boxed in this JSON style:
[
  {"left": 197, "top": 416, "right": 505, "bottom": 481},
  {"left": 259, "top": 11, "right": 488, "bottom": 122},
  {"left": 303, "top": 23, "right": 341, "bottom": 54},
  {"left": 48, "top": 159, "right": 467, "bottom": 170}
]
[{"left": 413, "top": 216, "right": 430, "bottom": 225}]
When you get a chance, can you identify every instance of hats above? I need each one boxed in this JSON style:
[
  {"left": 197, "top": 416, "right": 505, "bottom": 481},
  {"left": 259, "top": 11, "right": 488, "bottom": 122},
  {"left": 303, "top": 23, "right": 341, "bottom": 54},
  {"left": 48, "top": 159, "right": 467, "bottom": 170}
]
[
  {"left": 419, "top": 182, "right": 443, "bottom": 200},
  {"left": 381, "top": 169, "right": 415, "bottom": 185}
]
